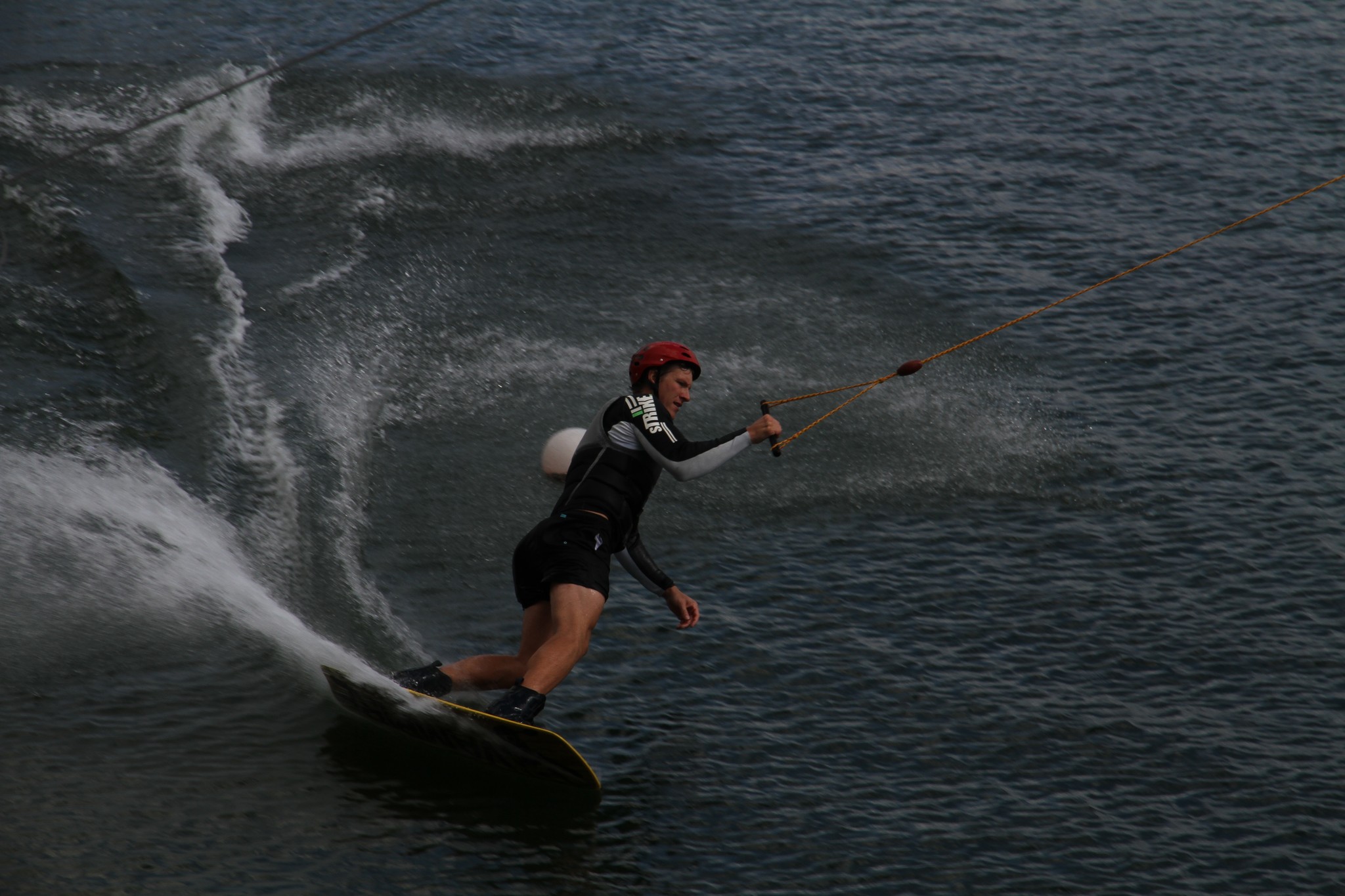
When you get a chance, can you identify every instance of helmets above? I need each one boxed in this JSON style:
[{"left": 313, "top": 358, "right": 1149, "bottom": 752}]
[{"left": 629, "top": 341, "right": 701, "bottom": 388}]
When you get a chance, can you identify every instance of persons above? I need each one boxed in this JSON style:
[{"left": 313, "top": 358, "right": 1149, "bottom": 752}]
[{"left": 395, "top": 341, "right": 784, "bottom": 722}]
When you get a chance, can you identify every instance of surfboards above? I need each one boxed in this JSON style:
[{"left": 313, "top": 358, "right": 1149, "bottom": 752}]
[{"left": 319, "top": 664, "right": 601, "bottom": 794}]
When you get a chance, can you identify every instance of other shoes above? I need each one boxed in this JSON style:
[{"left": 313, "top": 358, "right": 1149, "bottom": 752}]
[
  {"left": 391, "top": 660, "right": 452, "bottom": 697},
  {"left": 482, "top": 683, "right": 546, "bottom": 728}
]
